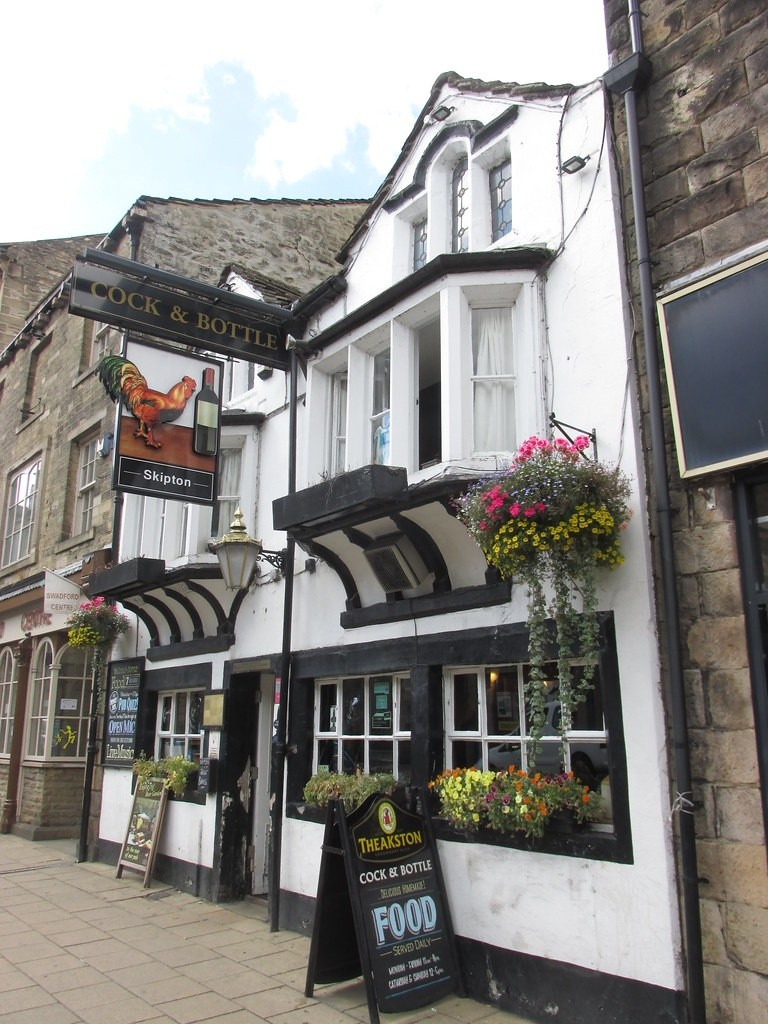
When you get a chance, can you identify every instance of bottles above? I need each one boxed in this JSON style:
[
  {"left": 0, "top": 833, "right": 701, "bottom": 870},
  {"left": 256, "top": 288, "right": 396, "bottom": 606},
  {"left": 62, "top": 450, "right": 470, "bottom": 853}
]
[{"left": 193, "top": 368, "right": 219, "bottom": 456}]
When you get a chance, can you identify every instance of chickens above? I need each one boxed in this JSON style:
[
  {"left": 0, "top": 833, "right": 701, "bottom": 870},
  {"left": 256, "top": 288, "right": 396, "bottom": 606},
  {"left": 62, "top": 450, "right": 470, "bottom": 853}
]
[{"left": 96, "top": 354, "right": 196, "bottom": 449}]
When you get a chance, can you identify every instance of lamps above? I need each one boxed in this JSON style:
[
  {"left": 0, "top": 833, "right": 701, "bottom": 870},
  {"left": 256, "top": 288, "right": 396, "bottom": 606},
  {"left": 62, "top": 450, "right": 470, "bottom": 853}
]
[
  {"left": 562, "top": 156, "right": 586, "bottom": 174},
  {"left": 432, "top": 105, "right": 452, "bottom": 122},
  {"left": 211, "top": 507, "right": 290, "bottom": 593}
]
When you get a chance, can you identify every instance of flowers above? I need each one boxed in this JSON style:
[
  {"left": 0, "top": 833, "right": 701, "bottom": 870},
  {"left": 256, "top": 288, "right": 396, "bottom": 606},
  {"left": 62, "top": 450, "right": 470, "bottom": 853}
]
[
  {"left": 64, "top": 597, "right": 130, "bottom": 648},
  {"left": 428, "top": 765, "right": 606, "bottom": 840},
  {"left": 452, "top": 435, "right": 631, "bottom": 766}
]
